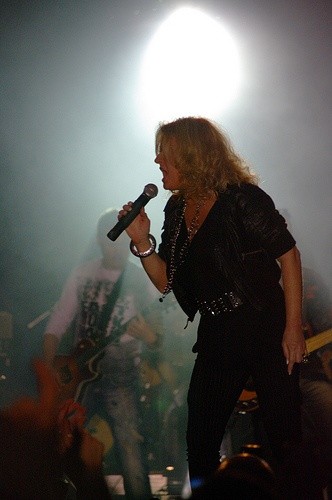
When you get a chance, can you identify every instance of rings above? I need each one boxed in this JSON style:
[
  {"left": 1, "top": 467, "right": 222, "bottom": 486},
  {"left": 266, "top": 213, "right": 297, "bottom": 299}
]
[{"left": 302, "top": 353, "right": 308, "bottom": 364}]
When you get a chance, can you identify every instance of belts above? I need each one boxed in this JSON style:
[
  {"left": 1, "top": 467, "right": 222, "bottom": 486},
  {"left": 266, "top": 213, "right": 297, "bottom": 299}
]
[{"left": 193, "top": 290, "right": 244, "bottom": 320}]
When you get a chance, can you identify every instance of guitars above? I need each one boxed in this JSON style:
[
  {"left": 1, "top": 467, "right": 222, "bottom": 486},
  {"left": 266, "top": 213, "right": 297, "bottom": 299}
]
[
  {"left": 36, "top": 292, "right": 178, "bottom": 407},
  {"left": 294, "top": 326, "right": 332, "bottom": 378}
]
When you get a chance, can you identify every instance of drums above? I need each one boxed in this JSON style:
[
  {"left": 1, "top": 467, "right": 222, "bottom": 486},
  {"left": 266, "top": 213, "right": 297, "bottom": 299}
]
[
  {"left": 235, "top": 390, "right": 259, "bottom": 429},
  {"left": 215, "top": 446, "right": 276, "bottom": 500}
]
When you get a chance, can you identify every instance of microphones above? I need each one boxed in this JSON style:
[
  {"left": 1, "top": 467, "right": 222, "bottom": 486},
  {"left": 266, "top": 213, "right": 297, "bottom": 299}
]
[{"left": 107, "top": 184, "right": 158, "bottom": 242}]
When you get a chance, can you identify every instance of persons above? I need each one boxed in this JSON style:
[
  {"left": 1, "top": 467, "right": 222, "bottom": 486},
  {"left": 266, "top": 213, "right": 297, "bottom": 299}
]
[
  {"left": 0, "top": 357, "right": 115, "bottom": 500},
  {"left": 117, "top": 116, "right": 310, "bottom": 499},
  {"left": 41, "top": 209, "right": 163, "bottom": 500},
  {"left": 230, "top": 210, "right": 331, "bottom": 500}
]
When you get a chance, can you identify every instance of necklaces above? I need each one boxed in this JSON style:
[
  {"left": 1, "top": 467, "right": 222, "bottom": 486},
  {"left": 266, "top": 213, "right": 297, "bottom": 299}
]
[{"left": 159, "top": 198, "right": 200, "bottom": 302}]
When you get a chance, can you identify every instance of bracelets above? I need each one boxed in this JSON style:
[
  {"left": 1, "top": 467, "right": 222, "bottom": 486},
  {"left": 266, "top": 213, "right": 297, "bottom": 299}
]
[
  {"left": 146, "top": 332, "right": 164, "bottom": 350},
  {"left": 130, "top": 234, "right": 156, "bottom": 257}
]
